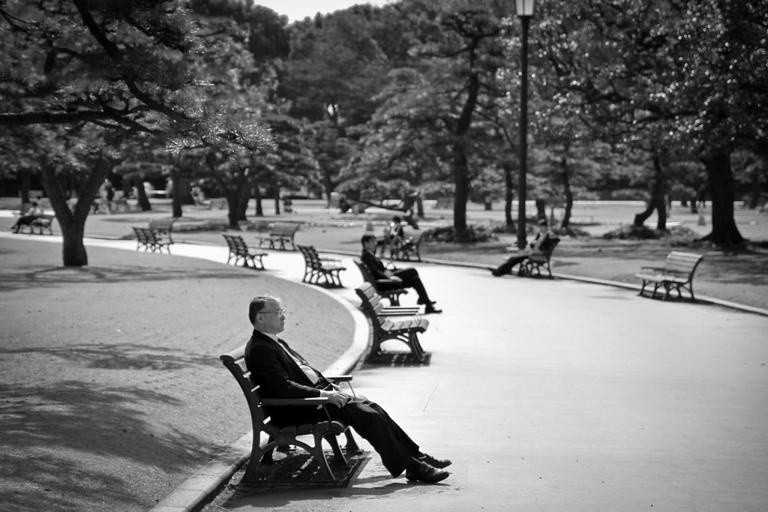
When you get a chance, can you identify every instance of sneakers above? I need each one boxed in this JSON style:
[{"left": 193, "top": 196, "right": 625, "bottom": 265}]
[{"left": 488, "top": 266, "right": 502, "bottom": 277}]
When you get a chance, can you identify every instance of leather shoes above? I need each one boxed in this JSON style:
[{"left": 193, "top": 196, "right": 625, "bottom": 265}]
[
  {"left": 406, "top": 460, "right": 449, "bottom": 484},
  {"left": 425, "top": 305, "right": 442, "bottom": 313},
  {"left": 417, "top": 451, "right": 452, "bottom": 468},
  {"left": 416, "top": 297, "right": 436, "bottom": 304}
]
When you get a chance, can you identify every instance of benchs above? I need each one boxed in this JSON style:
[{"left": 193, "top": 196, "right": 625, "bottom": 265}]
[
  {"left": 255, "top": 221, "right": 298, "bottom": 251},
  {"left": 520, "top": 234, "right": 559, "bottom": 279},
  {"left": 384, "top": 231, "right": 424, "bottom": 263},
  {"left": 223, "top": 234, "right": 268, "bottom": 271},
  {"left": 297, "top": 242, "right": 346, "bottom": 288},
  {"left": 221, "top": 341, "right": 371, "bottom": 492},
  {"left": 355, "top": 281, "right": 431, "bottom": 365},
  {"left": 633, "top": 249, "right": 704, "bottom": 301},
  {"left": 133, "top": 225, "right": 174, "bottom": 255}
]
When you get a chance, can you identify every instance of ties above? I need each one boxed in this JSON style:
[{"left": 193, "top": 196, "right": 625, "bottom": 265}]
[{"left": 278, "top": 339, "right": 327, "bottom": 388}]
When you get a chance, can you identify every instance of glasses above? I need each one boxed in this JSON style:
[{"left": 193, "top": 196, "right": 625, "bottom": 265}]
[{"left": 255, "top": 304, "right": 288, "bottom": 316}]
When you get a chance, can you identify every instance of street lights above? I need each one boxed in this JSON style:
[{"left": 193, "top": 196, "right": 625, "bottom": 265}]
[{"left": 512, "top": 0, "right": 538, "bottom": 247}]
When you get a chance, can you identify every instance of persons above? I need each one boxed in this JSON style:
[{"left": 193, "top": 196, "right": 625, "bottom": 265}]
[
  {"left": 486, "top": 218, "right": 551, "bottom": 277},
  {"left": 374, "top": 215, "right": 404, "bottom": 256},
  {"left": 10, "top": 202, "right": 42, "bottom": 234},
  {"left": 358, "top": 231, "right": 444, "bottom": 314},
  {"left": 242, "top": 291, "right": 454, "bottom": 484}
]
